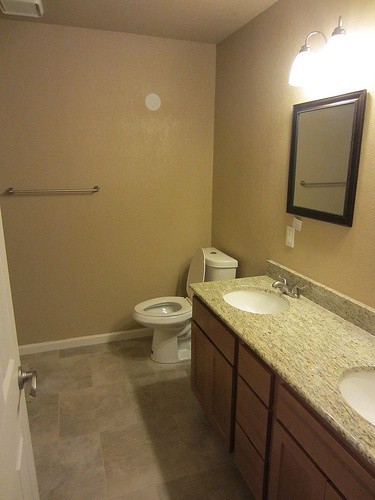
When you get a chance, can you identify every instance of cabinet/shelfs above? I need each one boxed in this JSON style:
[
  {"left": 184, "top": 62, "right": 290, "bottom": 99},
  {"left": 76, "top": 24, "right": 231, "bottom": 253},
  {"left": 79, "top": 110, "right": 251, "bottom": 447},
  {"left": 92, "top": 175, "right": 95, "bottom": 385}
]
[
  {"left": 191, "top": 295, "right": 237, "bottom": 453},
  {"left": 235, "top": 344, "right": 274, "bottom": 500},
  {"left": 267, "top": 418, "right": 346, "bottom": 500}
]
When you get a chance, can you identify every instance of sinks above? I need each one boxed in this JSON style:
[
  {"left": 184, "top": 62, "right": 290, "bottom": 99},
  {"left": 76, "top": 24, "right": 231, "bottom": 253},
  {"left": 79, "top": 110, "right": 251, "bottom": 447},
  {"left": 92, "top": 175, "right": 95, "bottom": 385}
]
[
  {"left": 222, "top": 286, "right": 290, "bottom": 315},
  {"left": 338, "top": 366, "right": 375, "bottom": 426}
]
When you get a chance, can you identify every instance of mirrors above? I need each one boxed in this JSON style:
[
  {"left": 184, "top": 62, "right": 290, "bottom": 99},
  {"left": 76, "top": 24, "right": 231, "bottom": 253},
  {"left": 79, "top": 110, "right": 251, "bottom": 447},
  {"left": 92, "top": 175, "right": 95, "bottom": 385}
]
[{"left": 287, "top": 89, "right": 367, "bottom": 228}]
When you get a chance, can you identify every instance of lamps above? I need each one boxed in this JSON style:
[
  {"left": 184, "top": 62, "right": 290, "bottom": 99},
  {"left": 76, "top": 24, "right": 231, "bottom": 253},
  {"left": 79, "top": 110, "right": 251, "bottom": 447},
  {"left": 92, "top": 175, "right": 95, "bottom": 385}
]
[{"left": 289, "top": 15, "right": 353, "bottom": 86}]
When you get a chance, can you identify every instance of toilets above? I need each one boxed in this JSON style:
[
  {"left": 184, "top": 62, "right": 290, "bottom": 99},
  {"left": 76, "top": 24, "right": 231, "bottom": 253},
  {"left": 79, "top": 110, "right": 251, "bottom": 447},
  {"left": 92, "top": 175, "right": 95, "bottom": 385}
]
[{"left": 132, "top": 247, "right": 238, "bottom": 363}]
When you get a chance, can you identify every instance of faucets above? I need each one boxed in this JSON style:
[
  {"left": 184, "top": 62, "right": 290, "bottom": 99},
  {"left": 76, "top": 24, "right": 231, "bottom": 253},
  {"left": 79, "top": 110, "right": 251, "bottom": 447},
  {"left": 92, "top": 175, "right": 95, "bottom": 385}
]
[{"left": 273, "top": 275, "right": 303, "bottom": 299}]
